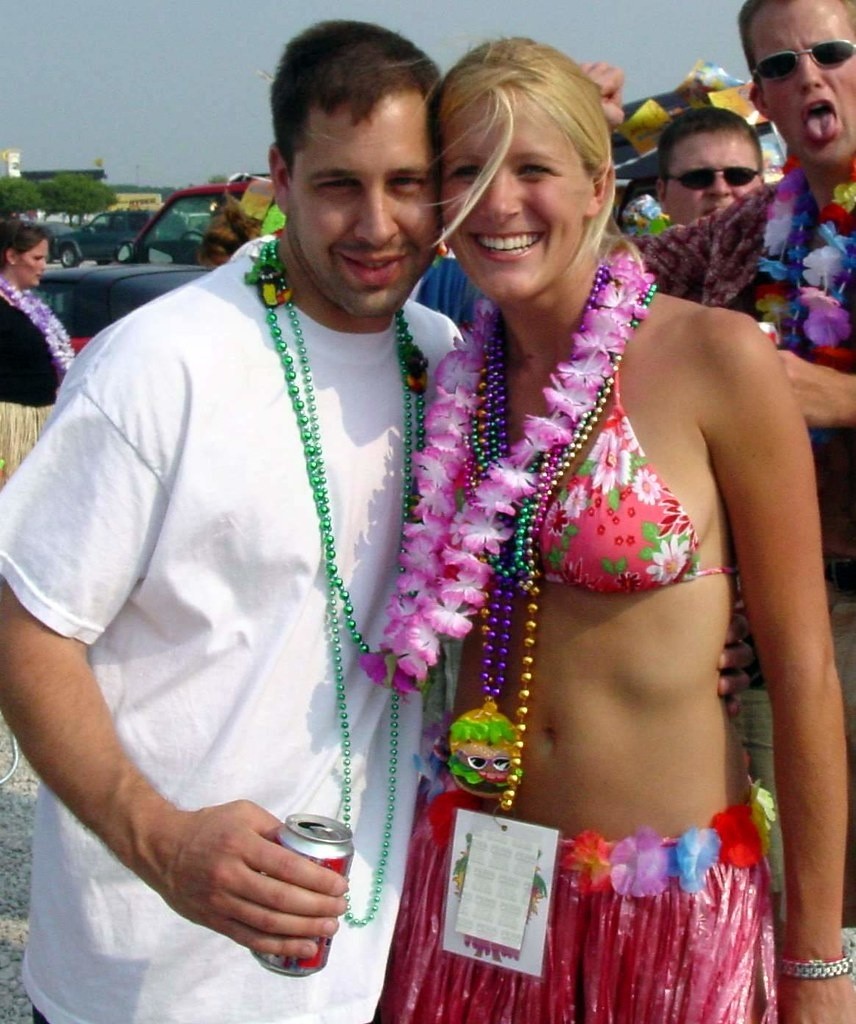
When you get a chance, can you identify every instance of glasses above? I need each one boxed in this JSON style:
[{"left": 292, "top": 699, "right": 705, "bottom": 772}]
[
  {"left": 667, "top": 165, "right": 762, "bottom": 190},
  {"left": 202, "top": 231, "right": 242, "bottom": 256},
  {"left": 752, "top": 40, "right": 856, "bottom": 80}
]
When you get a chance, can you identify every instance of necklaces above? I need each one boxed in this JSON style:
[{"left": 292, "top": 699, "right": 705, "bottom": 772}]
[
  {"left": 754, "top": 149, "right": 856, "bottom": 445},
  {"left": 0, "top": 276, "right": 77, "bottom": 379},
  {"left": 360, "top": 247, "right": 659, "bottom": 814},
  {"left": 244, "top": 239, "right": 433, "bottom": 926}
]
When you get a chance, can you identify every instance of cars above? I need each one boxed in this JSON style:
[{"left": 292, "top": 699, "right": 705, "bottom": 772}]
[
  {"left": 37, "top": 221, "right": 78, "bottom": 261},
  {"left": 23, "top": 172, "right": 271, "bottom": 356},
  {"left": 49, "top": 206, "right": 155, "bottom": 269},
  {"left": 117, "top": 178, "right": 256, "bottom": 269}
]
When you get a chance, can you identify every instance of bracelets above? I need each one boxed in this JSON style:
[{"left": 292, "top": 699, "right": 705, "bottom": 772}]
[{"left": 779, "top": 956, "right": 852, "bottom": 980}]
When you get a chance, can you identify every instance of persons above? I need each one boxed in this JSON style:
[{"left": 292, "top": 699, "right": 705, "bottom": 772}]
[
  {"left": 198, "top": 192, "right": 288, "bottom": 272},
  {"left": 0, "top": 219, "right": 76, "bottom": 488},
  {"left": 365, "top": 38, "right": 856, "bottom": 1024},
  {"left": 0, "top": 18, "right": 755, "bottom": 1024},
  {"left": 647, "top": 108, "right": 785, "bottom": 952},
  {"left": 574, "top": 1, "right": 856, "bottom": 984}
]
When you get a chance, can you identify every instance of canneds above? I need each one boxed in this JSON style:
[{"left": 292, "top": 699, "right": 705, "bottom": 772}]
[
  {"left": 756, "top": 321, "right": 781, "bottom": 349},
  {"left": 250, "top": 813, "right": 356, "bottom": 977}
]
